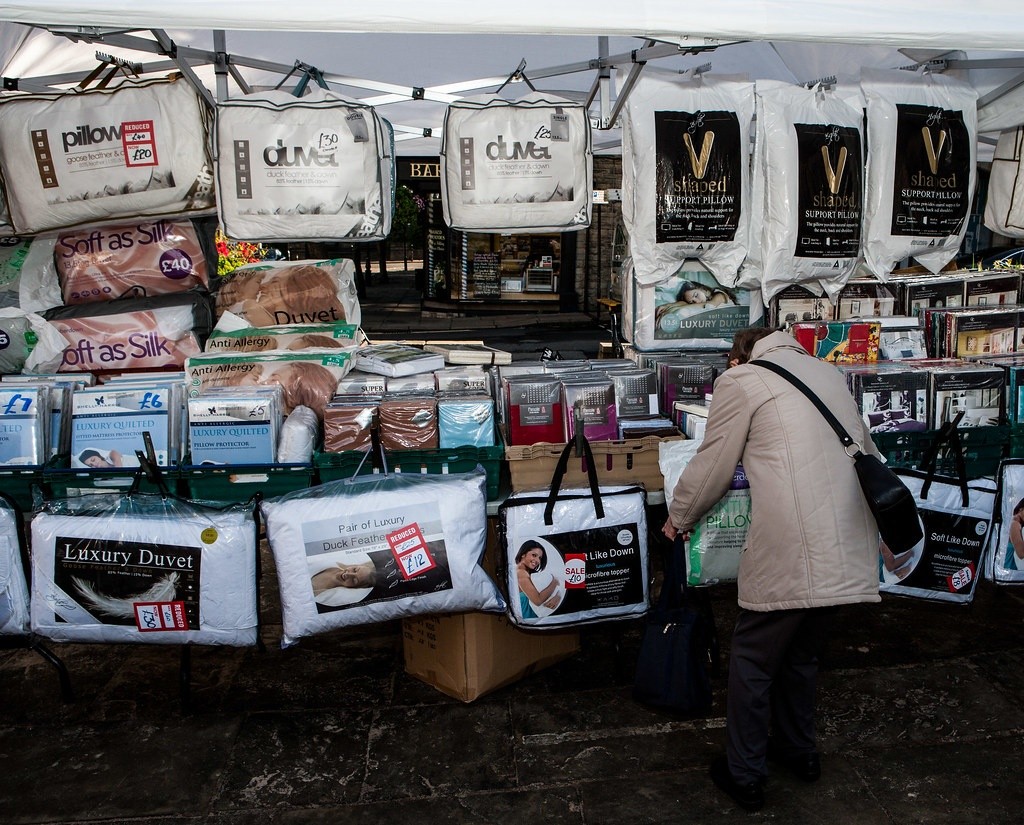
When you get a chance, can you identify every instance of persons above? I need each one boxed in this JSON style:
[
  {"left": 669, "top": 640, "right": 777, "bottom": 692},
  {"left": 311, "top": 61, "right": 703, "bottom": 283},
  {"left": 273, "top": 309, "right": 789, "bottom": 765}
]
[
  {"left": 654, "top": 282, "right": 734, "bottom": 330},
  {"left": 216, "top": 268, "right": 347, "bottom": 328},
  {"left": 661, "top": 326, "right": 883, "bottom": 810},
  {"left": 199, "top": 364, "right": 341, "bottom": 421},
  {"left": 1006, "top": 499, "right": 1024, "bottom": 571},
  {"left": 222, "top": 332, "right": 344, "bottom": 354},
  {"left": 517, "top": 540, "right": 561, "bottom": 620},
  {"left": 309, "top": 559, "right": 377, "bottom": 599},
  {"left": 80, "top": 450, "right": 143, "bottom": 467}
]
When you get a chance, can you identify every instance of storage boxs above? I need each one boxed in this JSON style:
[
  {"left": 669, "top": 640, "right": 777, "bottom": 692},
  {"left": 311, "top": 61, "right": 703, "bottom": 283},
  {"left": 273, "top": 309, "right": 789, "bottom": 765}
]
[
  {"left": 507, "top": 427, "right": 686, "bottom": 495},
  {"left": 399, "top": 608, "right": 581, "bottom": 702},
  {"left": 178, "top": 444, "right": 312, "bottom": 502},
  {"left": 598, "top": 341, "right": 613, "bottom": 359},
  {"left": 54, "top": 452, "right": 176, "bottom": 501},
  {"left": 0, "top": 458, "right": 51, "bottom": 507},
  {"left": 312, "top": 438, "right": 504, "bottom": 506}
]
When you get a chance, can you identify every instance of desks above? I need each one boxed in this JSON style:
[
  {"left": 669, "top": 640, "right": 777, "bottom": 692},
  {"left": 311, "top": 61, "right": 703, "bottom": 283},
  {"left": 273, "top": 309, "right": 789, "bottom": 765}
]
[{"left": 596, "top": 297, "right": 622, "bottom": 335}]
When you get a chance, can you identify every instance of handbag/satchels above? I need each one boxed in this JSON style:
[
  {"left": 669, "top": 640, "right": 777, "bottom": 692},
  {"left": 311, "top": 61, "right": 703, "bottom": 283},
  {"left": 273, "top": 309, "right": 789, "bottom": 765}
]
[
  {"left": 500, "top": 435, "right": 652, "bottom": 627},
  {"left": 844, "top": 441, "right": 923, "bottom": 556},
  {"left": 984, "top": 455, "right": 1024, "bottom": 586},
  {"left": 616, "top": 67, "right": 755, "bottom": 287},
  {"left": 738, "top": 79, "right": 868, "bottom": 307},
  {"left": 258, "top": 443, "right": 508, "bottom": 649},
  {"left": 631, "top": 611, "right": 713, "bottom": 718},
  {"left": 860, "top": 63, "right": 981, "bottom": 284},
  {"left": 0, "top": 55, "right": 219, "bottom": 237},
  {"left": 440, "top": 69, "right": 592, "bottom": 233},
  {"left": 30, "top": 460, "right": 259, "bottom": 646},
  {"left": 878, "top": 422, "right": 997, "bottom": 604},
  {"left": 210, "top": 66, "right": 395, "bottom": 243}
]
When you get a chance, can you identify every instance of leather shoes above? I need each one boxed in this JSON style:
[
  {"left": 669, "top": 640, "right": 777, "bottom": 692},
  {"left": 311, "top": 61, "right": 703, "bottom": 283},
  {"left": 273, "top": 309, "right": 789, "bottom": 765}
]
[
  {"left": 710, "top": 756, "right": 765, "bottom": 811},
  {"left": 771, "top": 745, "right": 821, "bottom": 781}
]
